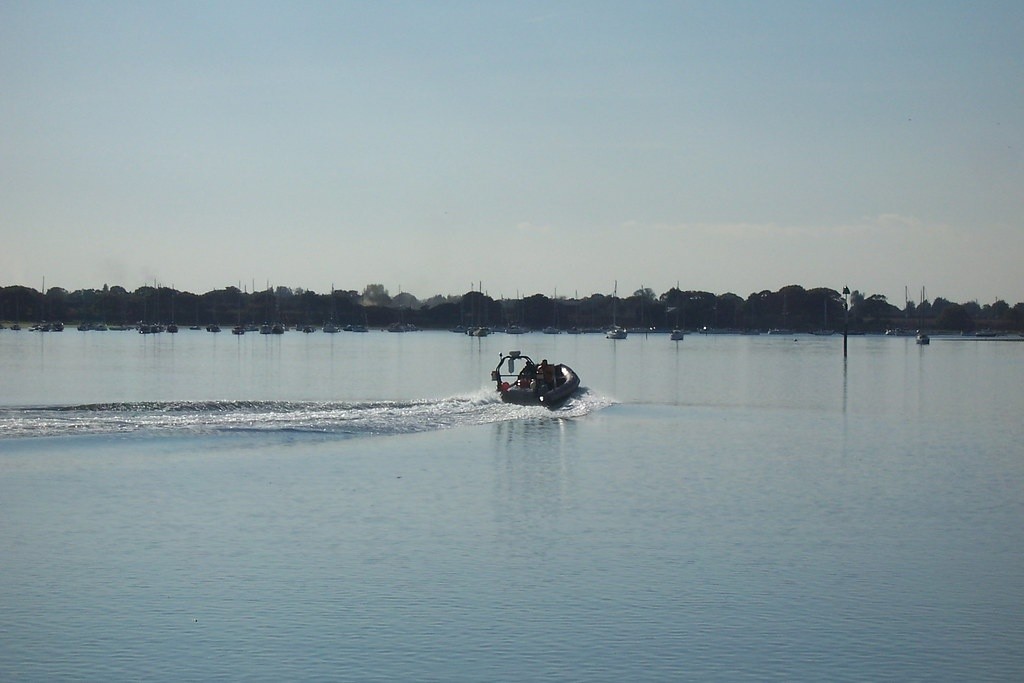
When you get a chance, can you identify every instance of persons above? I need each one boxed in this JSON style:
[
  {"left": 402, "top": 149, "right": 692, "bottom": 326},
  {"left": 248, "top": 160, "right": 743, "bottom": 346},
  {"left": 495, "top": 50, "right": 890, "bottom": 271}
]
[
  {"left": 519, "top": 361, "right": 539, "bottom": 383},
  {"left": 535, "top": 359, "right": 555, "bottom": 386}
]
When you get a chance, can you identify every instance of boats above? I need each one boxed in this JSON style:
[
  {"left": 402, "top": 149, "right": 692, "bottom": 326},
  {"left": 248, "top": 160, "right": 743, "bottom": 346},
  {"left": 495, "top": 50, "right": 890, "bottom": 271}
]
[{"left": 491, "top": 350, "right": 581, "bottom": 411}]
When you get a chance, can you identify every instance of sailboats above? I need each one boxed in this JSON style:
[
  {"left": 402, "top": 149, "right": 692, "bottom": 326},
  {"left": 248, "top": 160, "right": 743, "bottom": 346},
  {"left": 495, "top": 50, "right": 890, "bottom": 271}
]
[{"left": 2, "top": 279, "right": 1001, "bottom": 342}]
